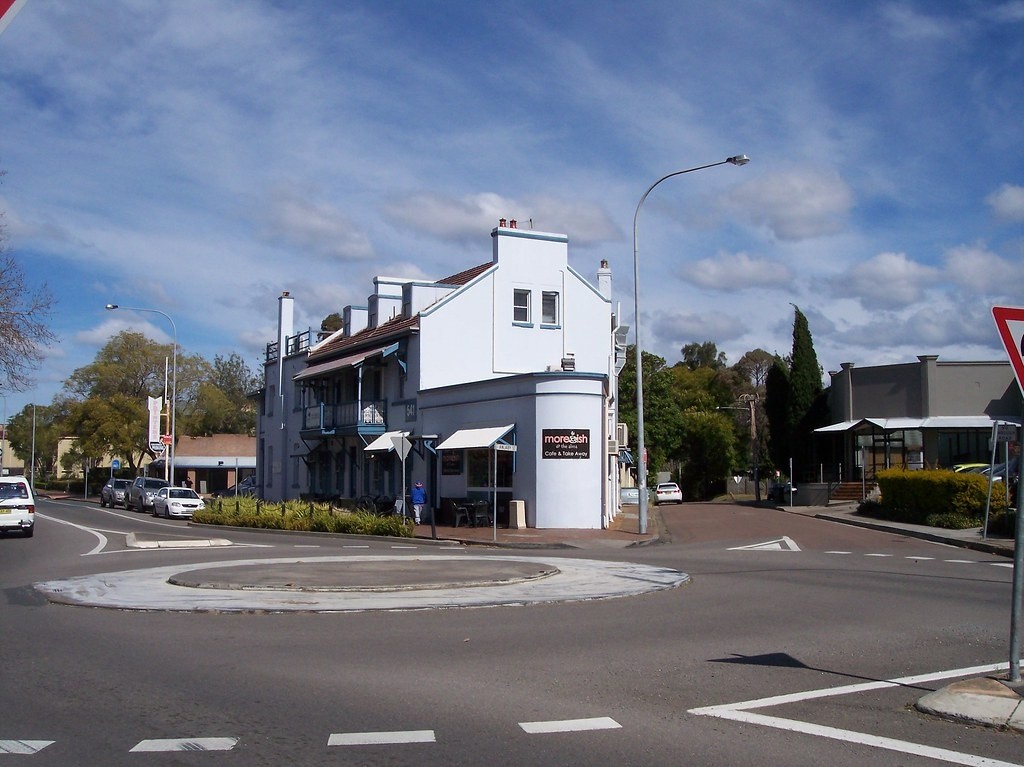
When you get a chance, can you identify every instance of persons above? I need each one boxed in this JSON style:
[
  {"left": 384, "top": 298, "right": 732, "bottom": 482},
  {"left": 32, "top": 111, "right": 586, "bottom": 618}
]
[
  {"left": 410, "top": 481, "right": 427, "bottom": 525},
  {"left": 185, "top": 477, "right": 193, "bottom": 488}
]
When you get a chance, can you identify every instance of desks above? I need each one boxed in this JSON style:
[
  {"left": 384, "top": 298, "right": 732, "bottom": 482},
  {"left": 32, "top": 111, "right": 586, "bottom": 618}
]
[{"left": 464, "top": 503, "right": 489, "bottom": 526}]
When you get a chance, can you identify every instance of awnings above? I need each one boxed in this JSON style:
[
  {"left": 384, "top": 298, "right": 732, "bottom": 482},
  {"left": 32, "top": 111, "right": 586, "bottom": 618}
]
[
  {"left": 363, "top": 431, "right": 410, "bottom": 452},
  {"left": 435, "top": 423, "right": 515, "bottom": 450}
]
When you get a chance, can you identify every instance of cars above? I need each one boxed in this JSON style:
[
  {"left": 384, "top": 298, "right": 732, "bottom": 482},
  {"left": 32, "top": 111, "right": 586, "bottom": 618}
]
[
  {"left": 0, "top": 476, "right": 36, "bottom": 539},
  {"left": 212, "top": 485, "right": 239, "bottom": 498},
  {"left": 787, "top": 482, "right": 797, "bottom": 493},
  {"left": 767, "top": 483, "right": 786, "bottom": 501},
  {"left": 152, "top": 487, "right": 207, "bottom": 520},
  {"left": 951, "top": 456, "right": 1019, "bottom": 491},
  {"left": 652, "top": 482, "right": 682, "bottom": 506},
  {"left": 124, "top": 476, "right": 172, "bottom": 513}
]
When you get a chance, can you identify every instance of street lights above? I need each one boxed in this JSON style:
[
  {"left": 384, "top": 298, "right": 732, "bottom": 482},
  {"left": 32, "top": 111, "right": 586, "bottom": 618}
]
[
  {"left": 633, "top": 154, "right": 751, "bottom": 535},
  {"left": 106, "top": 304, "right": 177, "bottom": 487}
]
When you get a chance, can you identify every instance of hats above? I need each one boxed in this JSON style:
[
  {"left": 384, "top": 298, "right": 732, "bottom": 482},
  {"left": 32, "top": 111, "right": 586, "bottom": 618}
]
[{"left": 416, "top": 480, "right": 423, "bottom": 484}]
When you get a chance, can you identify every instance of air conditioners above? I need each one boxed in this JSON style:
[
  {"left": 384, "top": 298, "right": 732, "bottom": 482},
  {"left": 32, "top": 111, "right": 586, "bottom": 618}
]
[{"left": 608, "top": 440, "right": 620, "bottom": 456}]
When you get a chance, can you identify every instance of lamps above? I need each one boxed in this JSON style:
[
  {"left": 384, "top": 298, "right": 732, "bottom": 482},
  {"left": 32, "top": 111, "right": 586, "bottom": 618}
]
[{"left": 561, "top": 358, "right": 576, "bottom": 371}]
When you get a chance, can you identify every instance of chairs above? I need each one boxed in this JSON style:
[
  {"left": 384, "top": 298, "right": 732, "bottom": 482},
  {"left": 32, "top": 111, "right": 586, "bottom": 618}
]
[{"left": 434, "top": 495, "right": 494, "bottom": 528}]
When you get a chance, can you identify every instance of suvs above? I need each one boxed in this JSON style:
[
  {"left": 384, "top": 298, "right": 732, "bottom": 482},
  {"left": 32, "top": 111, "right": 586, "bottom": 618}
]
[{"left": 100, "top": 478, "right": 134, "bottom": 509}]
